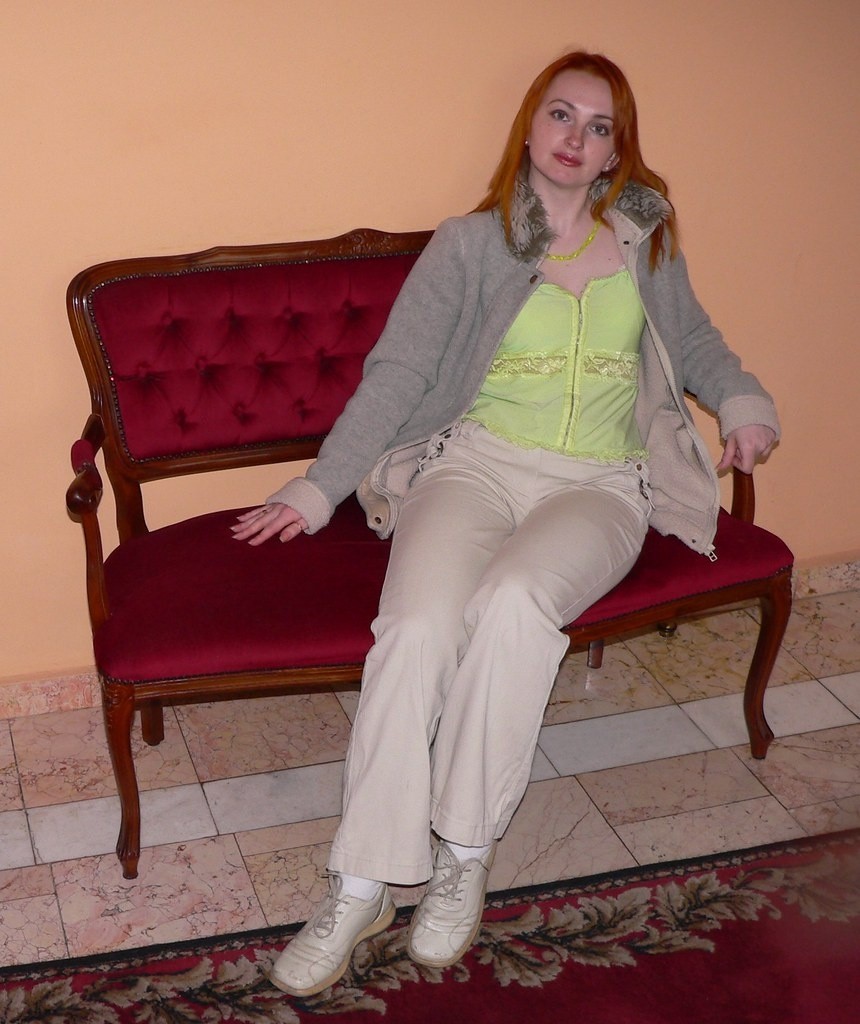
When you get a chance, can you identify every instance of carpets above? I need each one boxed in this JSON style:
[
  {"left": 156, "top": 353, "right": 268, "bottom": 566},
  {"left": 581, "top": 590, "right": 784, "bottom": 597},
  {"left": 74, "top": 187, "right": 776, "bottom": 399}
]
[{"left": 0, "top": 826, "right": 860, "bottom": 1024}]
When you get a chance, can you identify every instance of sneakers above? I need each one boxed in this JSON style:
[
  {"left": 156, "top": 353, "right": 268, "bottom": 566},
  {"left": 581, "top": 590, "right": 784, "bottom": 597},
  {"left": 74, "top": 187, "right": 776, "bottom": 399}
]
[
  {"left": 406, "top": 839, "right": 497, "bottom": 968},
  {"left": 268, "top": 871, "right": 397, "bottom": 997}
]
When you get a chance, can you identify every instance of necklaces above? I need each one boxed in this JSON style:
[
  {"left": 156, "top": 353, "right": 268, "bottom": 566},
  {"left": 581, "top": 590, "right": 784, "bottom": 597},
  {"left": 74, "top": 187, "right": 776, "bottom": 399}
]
[{"left": 545, "top": 221, "right": 602, "bottom": 261}]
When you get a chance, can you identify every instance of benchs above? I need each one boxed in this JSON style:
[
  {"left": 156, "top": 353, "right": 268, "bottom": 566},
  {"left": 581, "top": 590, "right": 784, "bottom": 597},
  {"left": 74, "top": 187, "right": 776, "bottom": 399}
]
[{"left": 67, "top": 228, "right": 795, "bottom": 879}]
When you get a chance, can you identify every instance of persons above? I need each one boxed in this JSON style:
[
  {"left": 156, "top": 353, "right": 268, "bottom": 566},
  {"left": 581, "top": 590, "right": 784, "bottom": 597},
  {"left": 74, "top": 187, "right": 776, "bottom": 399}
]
[{"left": 229, "top": 51, "right": 782, "bottom": 997}]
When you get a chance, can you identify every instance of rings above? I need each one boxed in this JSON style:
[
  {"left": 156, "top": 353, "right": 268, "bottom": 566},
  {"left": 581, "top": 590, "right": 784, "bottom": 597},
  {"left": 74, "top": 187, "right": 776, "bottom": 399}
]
[
  {"left": 295, "top": 521, "right": 303, "bottom": 531},
  {"left": 262, "top": 510, "right": 269, "bottom": 515}
]
